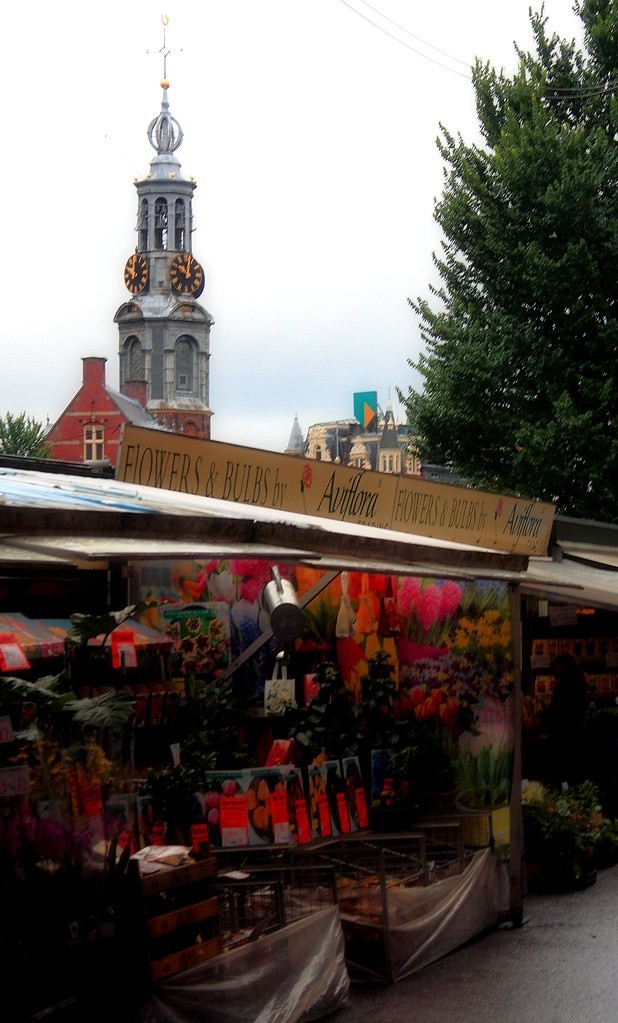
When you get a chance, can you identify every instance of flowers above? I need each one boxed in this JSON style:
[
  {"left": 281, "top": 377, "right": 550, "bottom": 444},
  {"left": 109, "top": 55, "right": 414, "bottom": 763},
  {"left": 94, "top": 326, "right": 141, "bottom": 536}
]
[
  {"left": 526, "top": 781, "right": 618, "bottom": 857},
  {"left": 13, "top": 814, "right": 96, "bottom": 885}
]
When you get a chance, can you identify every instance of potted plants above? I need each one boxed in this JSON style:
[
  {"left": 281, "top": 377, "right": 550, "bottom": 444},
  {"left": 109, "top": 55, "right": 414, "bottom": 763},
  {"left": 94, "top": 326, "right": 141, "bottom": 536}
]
[{"left": 452, "top": 743, "right": 513, "bottom": 849}]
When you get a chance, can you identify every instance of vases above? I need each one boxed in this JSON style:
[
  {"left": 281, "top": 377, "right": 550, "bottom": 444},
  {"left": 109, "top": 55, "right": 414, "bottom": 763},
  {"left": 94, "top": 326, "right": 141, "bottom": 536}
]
[{"left": 548, "top": 838, "right": 597, "bottom": 893}]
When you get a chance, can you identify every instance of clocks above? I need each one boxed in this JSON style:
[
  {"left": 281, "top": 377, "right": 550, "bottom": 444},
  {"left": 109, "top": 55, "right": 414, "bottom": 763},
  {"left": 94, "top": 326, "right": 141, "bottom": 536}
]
[
  {"left": 124, "top": 254, "right": 149, "bottom": 294},
  {"left": 169, "top": 254, "right": 203, "bottom": 294}
]
